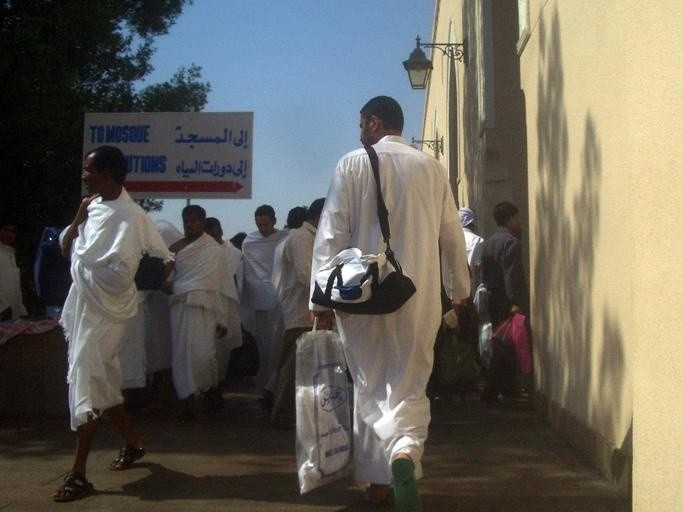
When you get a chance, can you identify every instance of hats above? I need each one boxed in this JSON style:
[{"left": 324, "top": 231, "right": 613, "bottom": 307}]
[{"left": 457, "top": 207, "right": 475, "bottom": 227}]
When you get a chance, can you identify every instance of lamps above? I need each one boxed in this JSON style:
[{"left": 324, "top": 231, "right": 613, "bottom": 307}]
[
  {"left": 409, "top": 136, "right": 444, "bottom": 157},
  {"left": 400, "top": 34, "right": 470, "bottom": 90}
]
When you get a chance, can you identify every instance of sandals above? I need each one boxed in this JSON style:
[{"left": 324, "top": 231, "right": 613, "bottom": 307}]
[
  {"left": 51, "top": 470, "right": 94, "bottom": 502},
  {"left": 106, "top": 442, "right": 145, "bottom": 471}
]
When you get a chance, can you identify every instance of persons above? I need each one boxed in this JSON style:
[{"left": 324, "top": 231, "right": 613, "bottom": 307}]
[{"left": 0, "top": 221, "right": 31, "bottom": 321}]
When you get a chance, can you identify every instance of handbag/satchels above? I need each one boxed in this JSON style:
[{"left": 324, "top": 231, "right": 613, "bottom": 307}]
[
  {"left": 433, "top": 333, "right": 485, "bottom": 387},
  {"left": 479, "top": 312, "right": 533, "bottom": 380},
  {"left": 33, "top": 223, "right": 167, "bottom": 308},
  {"left": 310, "top": 245, "right": 421, "bottom": 315}
]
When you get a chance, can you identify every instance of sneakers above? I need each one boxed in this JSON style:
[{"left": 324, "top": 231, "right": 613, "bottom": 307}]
[
  {"left": 390, "top": 452, "right": 424, "bottom": 511},
  {"left": 365, "top": 485, "right": 390, "bottom": 504}
]
